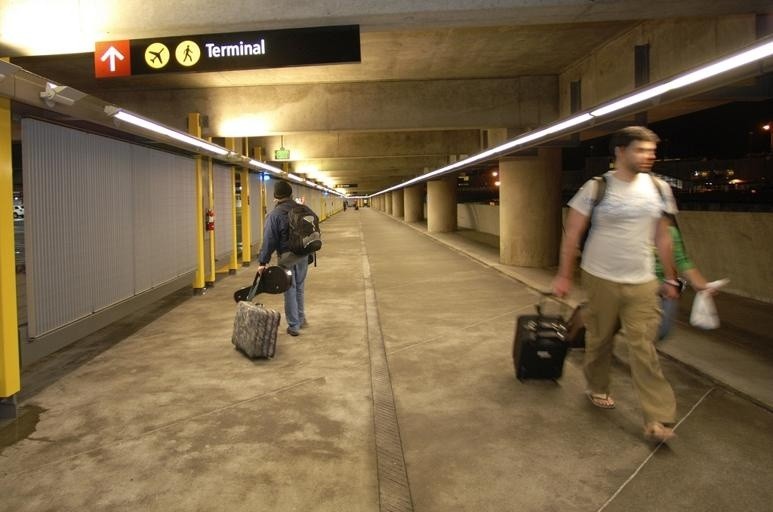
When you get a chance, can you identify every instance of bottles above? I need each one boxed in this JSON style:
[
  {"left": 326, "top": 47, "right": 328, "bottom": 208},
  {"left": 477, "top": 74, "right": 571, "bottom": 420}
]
[{"left": 274, "top": 181, "right": 292, "bottom": 199}]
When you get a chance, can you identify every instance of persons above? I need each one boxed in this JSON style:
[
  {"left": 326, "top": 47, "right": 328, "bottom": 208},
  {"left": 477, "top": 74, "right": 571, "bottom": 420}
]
[
  {"left": 550, "top": 123, "right": 682, "bottom": 444},
  {"left": 343, "top": 201, "right": 346, "bottom": 211},
  {"left": 655, "top": 218, "right": 720, "bottom": 346},
  {"left": 255, "top": 179, "right": 309, "bottom": 336}
]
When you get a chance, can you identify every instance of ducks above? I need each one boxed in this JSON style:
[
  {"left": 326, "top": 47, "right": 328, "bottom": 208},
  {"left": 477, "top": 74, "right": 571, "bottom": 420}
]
[
  {"left": 512, "top": 292, "right": 621, "bottom": 388},
  {"left": 231, "top": 300, "right": 281, "bottom": 360}
]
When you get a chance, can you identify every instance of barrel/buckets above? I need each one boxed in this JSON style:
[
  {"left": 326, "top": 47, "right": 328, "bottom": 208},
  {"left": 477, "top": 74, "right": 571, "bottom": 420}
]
[
  {"left": 643, "top": 422, "right": 675, "bottom": 442},
  {"left": 585, "top": 387, "right": 615, "bottom": 409}
]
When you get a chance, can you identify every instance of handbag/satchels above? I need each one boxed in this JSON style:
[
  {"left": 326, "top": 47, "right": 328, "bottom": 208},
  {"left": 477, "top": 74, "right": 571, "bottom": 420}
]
[{"left": 662, "top": 275, "right": 681, "bottom": 292}]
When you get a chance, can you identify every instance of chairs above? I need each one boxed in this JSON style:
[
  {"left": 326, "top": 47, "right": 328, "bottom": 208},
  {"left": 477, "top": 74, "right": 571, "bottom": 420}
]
[{"left": 287, "top": 318, "right": 305, "bottom": 337}]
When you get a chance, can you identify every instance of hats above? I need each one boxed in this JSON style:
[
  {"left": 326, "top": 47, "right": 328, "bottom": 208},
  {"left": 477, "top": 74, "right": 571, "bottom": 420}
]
[{"left": 14, "top": 205, "right": 24, "bottom": 218}]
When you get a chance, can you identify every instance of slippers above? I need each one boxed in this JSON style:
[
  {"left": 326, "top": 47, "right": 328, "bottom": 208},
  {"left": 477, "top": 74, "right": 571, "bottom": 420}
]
[
  {"left": 276, "top": 204, "right": 322, "bottom": 257},
  {"left": 561, "top": 175, "right": 664, "bottom": 267}
]
